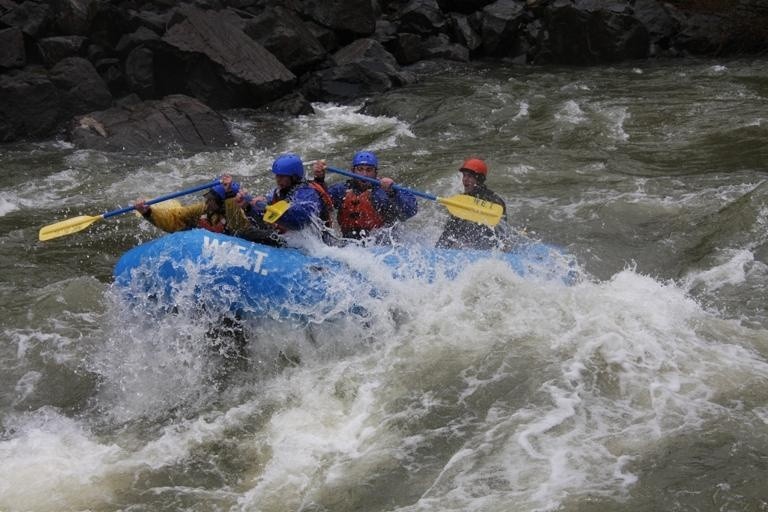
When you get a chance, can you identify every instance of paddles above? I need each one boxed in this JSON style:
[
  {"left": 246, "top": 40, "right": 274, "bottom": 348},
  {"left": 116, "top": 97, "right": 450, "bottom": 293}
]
[
  {"left": 323, "top": 164, "right": 503, "bottom": 228},
  {"left": 244, "top": 194, "right": 290, "bottom": 223},
  {"left": 39, "top": 179, "right": 222, "bottom": 243}
]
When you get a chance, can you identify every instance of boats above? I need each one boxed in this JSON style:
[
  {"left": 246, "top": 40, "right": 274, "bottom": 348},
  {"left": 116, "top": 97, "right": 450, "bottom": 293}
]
[{"left": 110, "top": 225, "right": 584, "bottom": 346}]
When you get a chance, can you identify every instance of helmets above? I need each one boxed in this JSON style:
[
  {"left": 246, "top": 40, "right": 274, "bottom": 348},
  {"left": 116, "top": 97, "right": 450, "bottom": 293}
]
[
  {"left": 459, "top": 158, "right": 488, "bottom": 183},
  {"left": 212, "top": 175, "right": 239, "bottom": 198},
  {"left": 272, "top": 156, "right": 304, "bottom": 178},
  {"left": 353, "top": 153, "right": 378, "bottom": 168}
]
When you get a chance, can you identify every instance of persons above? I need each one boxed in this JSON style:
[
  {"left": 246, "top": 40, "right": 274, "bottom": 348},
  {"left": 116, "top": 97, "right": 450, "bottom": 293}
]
[
  {"left": 134, "top": 152, "right": 418, "bottom": 247},
  {"left": 434, "top": 159, "right": 507, "bottom": 250}
]
